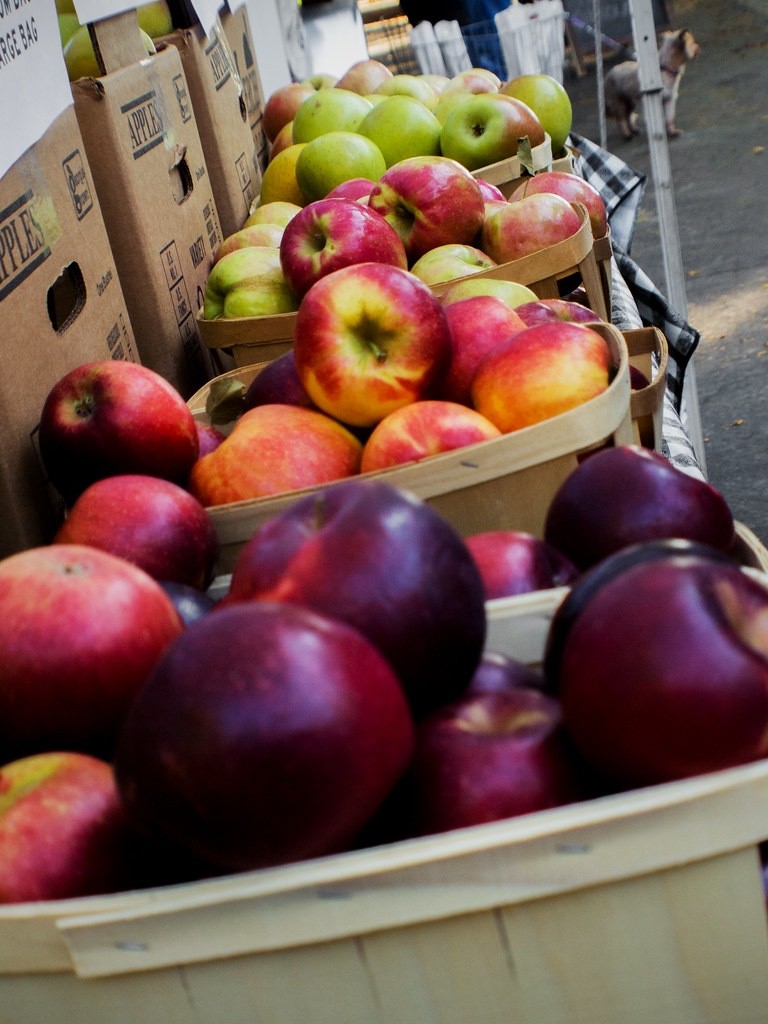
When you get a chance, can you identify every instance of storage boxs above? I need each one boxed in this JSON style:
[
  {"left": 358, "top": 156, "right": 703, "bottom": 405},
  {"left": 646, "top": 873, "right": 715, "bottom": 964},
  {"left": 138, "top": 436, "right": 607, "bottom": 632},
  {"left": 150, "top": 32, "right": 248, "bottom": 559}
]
[
  {"left": 221, "top": 4, "right": 267, "bottom": 166},
  {"left": 151, "top": 24, "right": 262, "bottom": 239},
  {"left": 0, "top": 104, "right": 143, "bottom": 552},
  {"left": 69, "top": 46, "right": 226, "bottom": 388}
]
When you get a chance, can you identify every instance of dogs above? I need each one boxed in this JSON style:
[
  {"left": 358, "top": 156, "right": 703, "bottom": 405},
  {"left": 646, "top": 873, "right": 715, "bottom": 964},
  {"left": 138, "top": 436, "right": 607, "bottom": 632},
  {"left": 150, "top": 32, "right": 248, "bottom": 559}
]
[{"left": 606, "top": 29, "right": 699, "bottom": 142}]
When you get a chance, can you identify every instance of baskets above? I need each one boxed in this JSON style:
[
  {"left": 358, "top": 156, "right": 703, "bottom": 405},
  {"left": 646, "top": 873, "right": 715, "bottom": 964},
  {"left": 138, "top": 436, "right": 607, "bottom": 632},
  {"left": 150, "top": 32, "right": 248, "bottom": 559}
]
[
  {"left": 247, "top": 135, "right": 553, "bottom": 204},
  {"left": 617, "top": 321, "right": 668, "bottom": 453},
  {"left": 195, "top": 194, "right": 612, "bottom": 371},
  {"left": 1, "top": 587, "right": 767, "bottom": 1024},
  {"left": 172, "top": 322, "right": 644, "bottom": 586}
]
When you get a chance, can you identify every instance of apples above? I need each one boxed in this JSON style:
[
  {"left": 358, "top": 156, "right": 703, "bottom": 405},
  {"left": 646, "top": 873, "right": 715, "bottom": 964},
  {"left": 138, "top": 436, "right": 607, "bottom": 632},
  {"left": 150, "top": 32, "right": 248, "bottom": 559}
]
[{"left": 0, "top": 0, "right": 768, "bottom": 907}]
